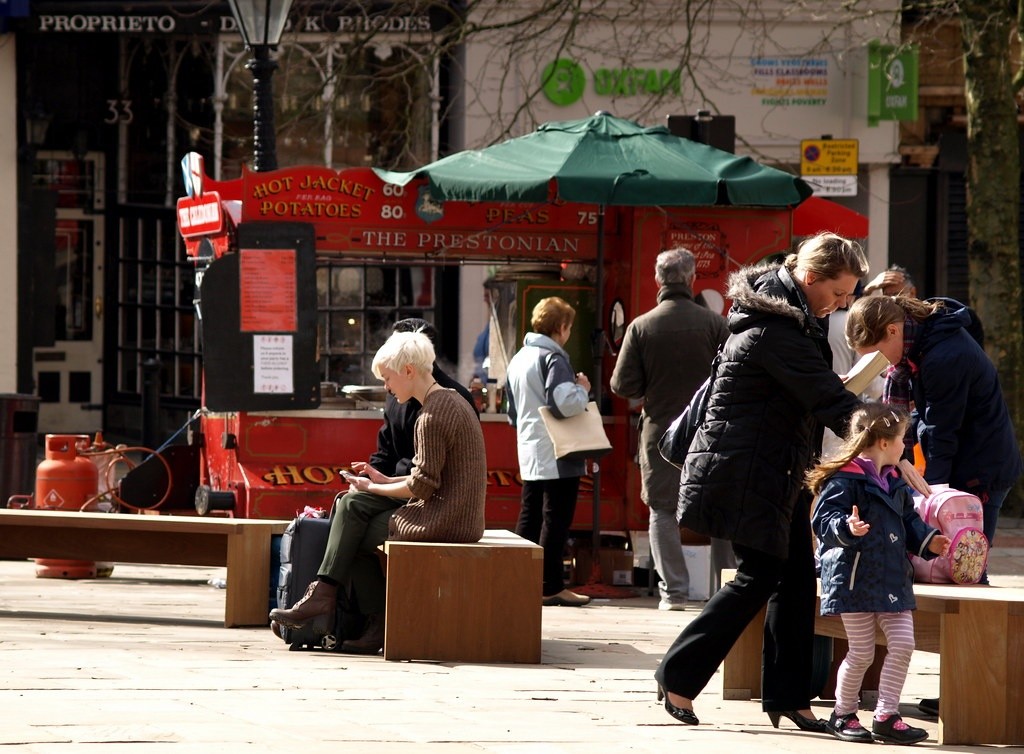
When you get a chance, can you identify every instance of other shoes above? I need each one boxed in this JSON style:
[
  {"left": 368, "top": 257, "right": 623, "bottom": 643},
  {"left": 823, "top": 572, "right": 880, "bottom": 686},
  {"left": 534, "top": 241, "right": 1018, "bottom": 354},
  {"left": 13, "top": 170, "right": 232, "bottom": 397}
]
[
  {"left": 659, "top": 599, "right": 684, "bottom": 610},
  {"left": 918, "top": 699, "right": 938, "bottom": 715},
  {"left": 542, "top": 592, "right": 590, "bottom": 606}
]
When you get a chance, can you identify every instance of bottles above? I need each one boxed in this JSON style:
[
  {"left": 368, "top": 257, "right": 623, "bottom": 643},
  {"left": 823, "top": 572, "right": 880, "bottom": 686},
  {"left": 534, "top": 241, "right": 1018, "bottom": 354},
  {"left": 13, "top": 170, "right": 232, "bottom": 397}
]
[
  {"left": 485, "top": 379, "right": 496, "bottom": 413},
  {"left": 471, "top": 375, "right": 482, "bottom": 411}
]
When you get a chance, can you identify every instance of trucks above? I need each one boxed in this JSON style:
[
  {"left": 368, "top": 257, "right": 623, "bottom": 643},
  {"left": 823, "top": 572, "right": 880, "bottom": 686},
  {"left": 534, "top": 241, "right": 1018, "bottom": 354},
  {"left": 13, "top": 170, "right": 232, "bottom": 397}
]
[{"left": 176, "top": 109, "right": 870, "bottom": 532}]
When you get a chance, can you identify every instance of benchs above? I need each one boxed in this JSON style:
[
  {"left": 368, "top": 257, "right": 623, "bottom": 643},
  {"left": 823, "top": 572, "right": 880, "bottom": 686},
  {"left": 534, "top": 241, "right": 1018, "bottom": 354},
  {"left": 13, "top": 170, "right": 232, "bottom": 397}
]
[
  {"left": 721, "top": 569, "right": 1024, "bottom": 747},
  {"left": 0, "top": 509, "right": 292, "bottom": 628},
  {"left": 377, "top": 529, "right": 544, "bottom": 666}
]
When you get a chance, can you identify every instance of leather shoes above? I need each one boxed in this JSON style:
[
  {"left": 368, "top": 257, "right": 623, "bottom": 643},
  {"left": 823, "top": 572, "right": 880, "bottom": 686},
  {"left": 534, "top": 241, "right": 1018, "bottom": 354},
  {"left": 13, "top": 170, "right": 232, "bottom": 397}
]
[
  {"left": 870, "top": 713, "right": 928, "bottom": 744},
  {"left": 825, "top": 711, "right": 871, "bottom": 741}
]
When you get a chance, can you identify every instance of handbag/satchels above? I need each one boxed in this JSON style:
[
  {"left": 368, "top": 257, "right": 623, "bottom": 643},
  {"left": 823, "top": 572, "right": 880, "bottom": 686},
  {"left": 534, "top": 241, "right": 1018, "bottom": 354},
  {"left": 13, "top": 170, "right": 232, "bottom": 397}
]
[
  {"left": 537, "top": 352, "right": 612, "bottom": 461},
  {"left": 657, "top": 344, "right": 723, "bottom": 470}
]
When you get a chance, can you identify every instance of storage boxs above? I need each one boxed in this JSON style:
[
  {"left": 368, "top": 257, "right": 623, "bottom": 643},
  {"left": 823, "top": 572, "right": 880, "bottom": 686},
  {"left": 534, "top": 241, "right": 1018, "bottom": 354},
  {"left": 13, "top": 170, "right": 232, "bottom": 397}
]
[
  {"left": 598, "top": 549, "right": 633, "bottom": 585},
  {"left": 680, "top": 545, "right": 716, "bottom": 601}
]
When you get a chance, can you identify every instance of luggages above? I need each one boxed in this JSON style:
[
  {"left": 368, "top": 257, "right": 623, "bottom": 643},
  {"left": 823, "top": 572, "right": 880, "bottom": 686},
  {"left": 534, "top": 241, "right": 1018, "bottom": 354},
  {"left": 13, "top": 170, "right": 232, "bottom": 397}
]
[{"left": 277, "top": 490, "right": 348, "bottom": 650}]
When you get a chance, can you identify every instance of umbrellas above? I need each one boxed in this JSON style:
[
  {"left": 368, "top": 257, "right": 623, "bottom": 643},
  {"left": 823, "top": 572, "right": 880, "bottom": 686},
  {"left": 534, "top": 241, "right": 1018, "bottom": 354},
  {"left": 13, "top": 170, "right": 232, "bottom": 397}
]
[{"left": 373, "top": 107, "right": 814, "bottom": 587}]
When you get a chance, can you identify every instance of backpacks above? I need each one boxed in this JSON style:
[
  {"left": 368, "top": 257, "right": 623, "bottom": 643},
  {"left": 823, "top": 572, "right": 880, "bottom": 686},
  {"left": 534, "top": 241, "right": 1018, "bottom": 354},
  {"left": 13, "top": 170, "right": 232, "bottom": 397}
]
[{"left": 904, "top": 483, "right": 988, "bottom": 586}]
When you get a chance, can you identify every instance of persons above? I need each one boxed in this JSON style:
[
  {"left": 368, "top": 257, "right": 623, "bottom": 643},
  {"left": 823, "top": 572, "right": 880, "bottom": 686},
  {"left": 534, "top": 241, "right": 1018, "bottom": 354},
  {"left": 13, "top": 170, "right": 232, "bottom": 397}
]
[
  {"left": 654, "top": 235, "right": 1018, "bottom": 743},
  {"left": 506, "top": 296, "right": 592, "bottom": 608},
  {"left": 268, "top": 316, "right": 487, "bottom": 650},
  {"left": 469, "top": 275, "right": 495, "bottom": 390},
  {"left": 610, "top": 246, "right": 737, "bottom": 612}
]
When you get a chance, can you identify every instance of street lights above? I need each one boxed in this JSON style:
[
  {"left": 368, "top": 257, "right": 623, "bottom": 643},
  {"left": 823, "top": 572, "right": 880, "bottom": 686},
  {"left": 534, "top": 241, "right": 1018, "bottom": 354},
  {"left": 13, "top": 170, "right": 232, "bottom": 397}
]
[{"left": 230, "top": 0, "right": 297, "bottom": 173}]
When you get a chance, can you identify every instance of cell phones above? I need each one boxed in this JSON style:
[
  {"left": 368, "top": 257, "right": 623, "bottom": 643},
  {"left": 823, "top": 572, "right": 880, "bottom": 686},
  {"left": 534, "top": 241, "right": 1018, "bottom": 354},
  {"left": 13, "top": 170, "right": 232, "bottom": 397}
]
[{"left": 339, "top": 470, "right": 354, "bottom": 479}]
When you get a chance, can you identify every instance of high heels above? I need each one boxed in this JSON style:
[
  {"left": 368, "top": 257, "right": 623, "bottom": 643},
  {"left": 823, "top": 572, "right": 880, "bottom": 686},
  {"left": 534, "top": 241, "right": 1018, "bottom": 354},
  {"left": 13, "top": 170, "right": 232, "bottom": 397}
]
[
  {"left": 767, "top": 710, "right": 829, "bottom": 732},
  {"left": 269, "top": 581, "right": 335, "bottom": 631},
  {"left": 658, "top": 682, "right": 699, "bottom": 725},
  {"left": 342, "top": 610, "right": 384, "bottom": 652}
]
